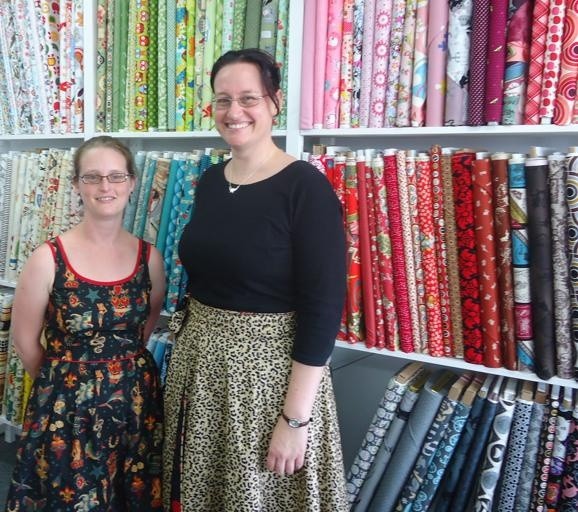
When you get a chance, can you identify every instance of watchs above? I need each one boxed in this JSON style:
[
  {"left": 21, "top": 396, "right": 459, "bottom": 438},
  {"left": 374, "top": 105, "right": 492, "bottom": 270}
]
[{"left": 280, "top": 410, "right": 316, "bottom": 430}]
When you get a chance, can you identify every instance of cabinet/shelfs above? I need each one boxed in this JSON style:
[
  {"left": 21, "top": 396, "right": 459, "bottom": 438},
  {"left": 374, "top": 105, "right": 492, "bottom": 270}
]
[{"left": 0, "top": 0, "right": 578, "bottom": 443}]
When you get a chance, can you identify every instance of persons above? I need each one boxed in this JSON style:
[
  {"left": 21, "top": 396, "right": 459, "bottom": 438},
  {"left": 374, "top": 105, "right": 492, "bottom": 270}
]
[
  {"left": 160, "top": 47, "right": 350, "bottom": 511},
  {"left": 5, "top": 134, "right": 166, "bottom": 511}
]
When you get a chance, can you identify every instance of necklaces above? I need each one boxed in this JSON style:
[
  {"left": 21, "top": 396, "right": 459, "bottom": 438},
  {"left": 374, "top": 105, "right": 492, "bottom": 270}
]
[{"left": 229, "top": 147, "right": 279, "bottom": 194}]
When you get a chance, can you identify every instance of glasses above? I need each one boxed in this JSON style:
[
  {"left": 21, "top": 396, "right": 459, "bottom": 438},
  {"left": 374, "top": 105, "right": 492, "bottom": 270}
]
[
  {"left": 77, "top": 172, "right": 132, "bottom": 183},
  {"left": 211, "top": 95, "right": 271, "bottom": 110}
]
